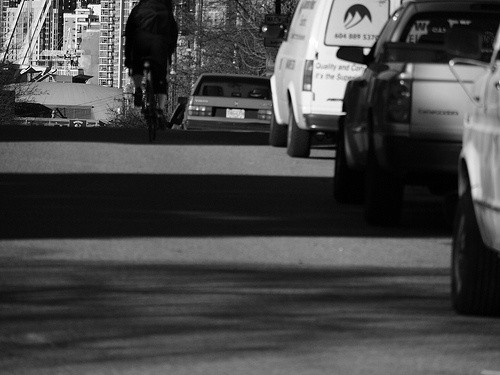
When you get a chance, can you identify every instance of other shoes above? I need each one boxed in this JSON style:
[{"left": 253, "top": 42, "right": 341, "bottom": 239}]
[
  {"left": 157, "top": 108, "right": 167, "bottom": 132},
  {"left": 134, "top": 87, "right": 143, "bottom": 107}
]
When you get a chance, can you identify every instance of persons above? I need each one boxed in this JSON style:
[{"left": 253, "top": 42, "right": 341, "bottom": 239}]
[{"left": 122, "top": 0, "right": 179, "bottom": 121}]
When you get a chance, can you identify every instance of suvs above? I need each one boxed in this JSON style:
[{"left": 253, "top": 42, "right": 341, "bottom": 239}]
[{"left": 259, "top": 0, "right": 471, "bottom": 159}]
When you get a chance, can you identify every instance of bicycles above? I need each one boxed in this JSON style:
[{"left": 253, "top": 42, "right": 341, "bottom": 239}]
[{"left": 129, "top": 64, "right": 164, "bottom": 143}]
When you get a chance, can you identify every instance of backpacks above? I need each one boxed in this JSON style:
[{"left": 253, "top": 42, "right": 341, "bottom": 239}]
[{"left": 134, "top": 0, "right": 178, "bottom": 58}]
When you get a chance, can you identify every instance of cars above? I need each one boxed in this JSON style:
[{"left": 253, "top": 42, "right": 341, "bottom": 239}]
[
  {"left": 177, "top": 73, "right": 273, "bottom": 143},
  {"left": 333, "top": 1, "right": 499, "bottom": 221},
  {"left": 451, "top": 21, "right": 500, "bottom": 314}
]
